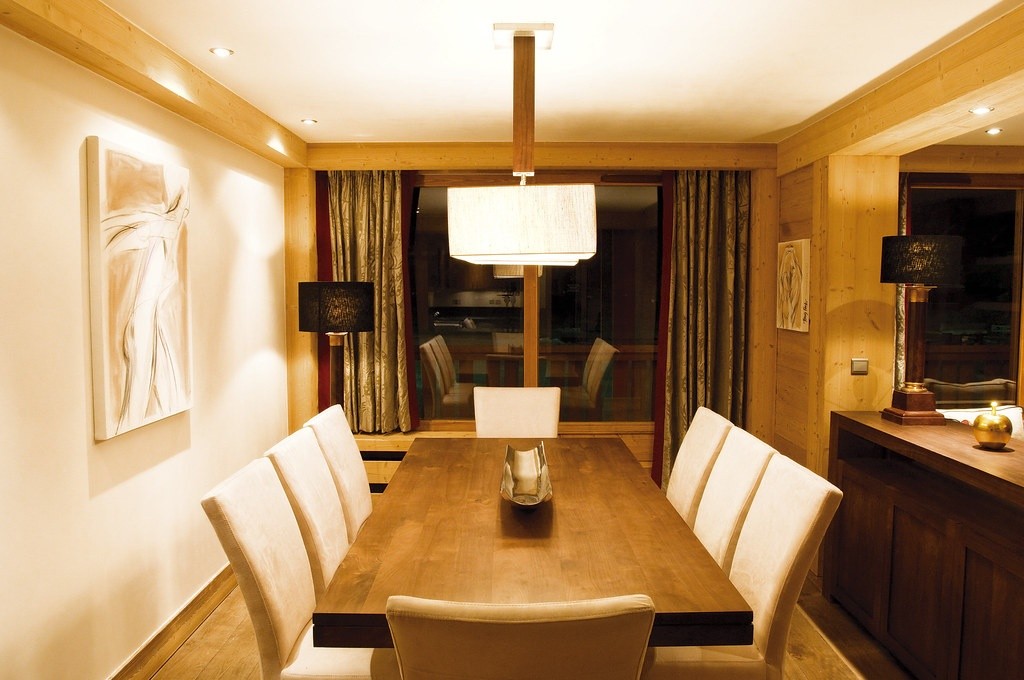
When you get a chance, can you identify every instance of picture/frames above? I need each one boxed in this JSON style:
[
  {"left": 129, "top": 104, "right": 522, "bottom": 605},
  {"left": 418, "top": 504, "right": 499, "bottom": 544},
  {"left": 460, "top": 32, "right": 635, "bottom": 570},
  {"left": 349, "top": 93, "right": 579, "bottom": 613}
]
[{"left": 89, "top": 133, "right": 196, "bottom": 439}]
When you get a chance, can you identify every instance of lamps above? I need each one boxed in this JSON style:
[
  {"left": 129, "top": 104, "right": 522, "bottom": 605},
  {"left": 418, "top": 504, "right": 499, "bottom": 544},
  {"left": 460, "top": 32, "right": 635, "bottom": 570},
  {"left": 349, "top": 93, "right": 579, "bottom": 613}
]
[
  {"left": 494, "top": 265, "right": 544, "bottom": 278},
  {"left": 298, "top": 281, "right": 374, "bottom": 411},
  {"left": 449, "top": 20, "right": 596, "bottom": 265},
  {"left": 879, "top": 235, "right": 960, "bottom": 426}
]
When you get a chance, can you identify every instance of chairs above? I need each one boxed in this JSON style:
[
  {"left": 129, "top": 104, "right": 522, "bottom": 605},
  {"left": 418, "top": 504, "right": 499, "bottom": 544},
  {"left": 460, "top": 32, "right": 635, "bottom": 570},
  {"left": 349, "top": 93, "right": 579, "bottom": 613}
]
[
  {"left": 420, "top": 334, "right": 475, "bottom": 419},
  {"left": 471, "top": 388, "right": 561, "bottom": 438},
  {"left": 642, "top": 406, "right": 844, "bottom": 680},
  {"left": 553, "top": 338, "right": 621, "bottom": 421},
  {"left": 200, "top": 402, "right": 403, "bottom": 680},
  {"left": 494, "top": 332, "right": 528, "bottom": 354},
  {"left": 384, "top": 594, "right": 655, "bottom": 680}
]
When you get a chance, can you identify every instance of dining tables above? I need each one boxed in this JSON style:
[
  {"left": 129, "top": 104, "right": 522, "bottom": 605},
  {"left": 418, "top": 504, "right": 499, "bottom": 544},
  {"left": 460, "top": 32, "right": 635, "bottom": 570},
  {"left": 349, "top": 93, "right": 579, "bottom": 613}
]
[{"left": 313, "top": 437, "right": 756, "bottom": 648}]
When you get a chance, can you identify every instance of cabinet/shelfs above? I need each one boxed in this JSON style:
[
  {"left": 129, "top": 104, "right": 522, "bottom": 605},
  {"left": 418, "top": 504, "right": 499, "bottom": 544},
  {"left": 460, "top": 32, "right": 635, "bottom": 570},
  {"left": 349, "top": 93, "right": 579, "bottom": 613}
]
[{"left": 821, "top": 409, "right": 1024, "bottom": 680}]
